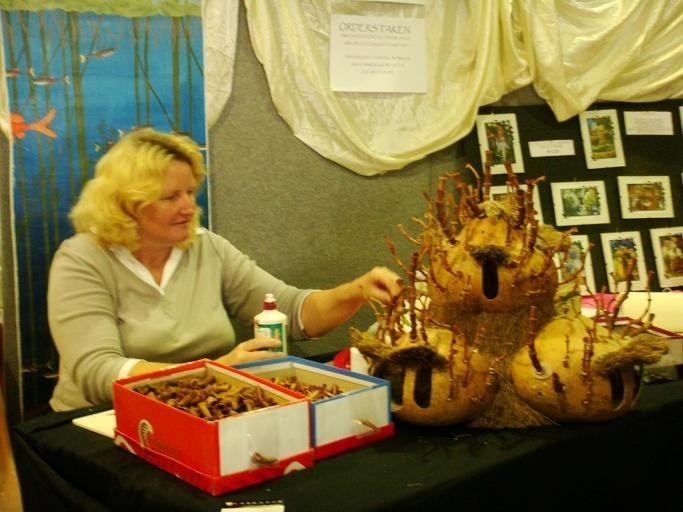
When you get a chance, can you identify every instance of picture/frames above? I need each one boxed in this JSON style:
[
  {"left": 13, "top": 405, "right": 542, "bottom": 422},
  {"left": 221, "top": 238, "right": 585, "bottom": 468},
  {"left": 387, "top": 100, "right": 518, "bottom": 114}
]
[
  {"left": 650, "top": 226, "right": 683, "bottom": 287},
  {"left": 551, "top": 234, "right": 598, "bottom": 296},
  {"left": 475, "top": 112, "right": 525, "bottom": 175},
  {"left": 617, "top": 175, "right": 675, "bottom": 220},
  {"left": 550, "top": 179, "right": 611, "bottom": 228},
  {"left": 578, "top": 109, "right": 627, "bottom": 168},
  {"left": 487, "top": 184, "right": 544, "bottom": 227},
  {"left": 599, "top": 231, "right": 649, "bottom": 292}
]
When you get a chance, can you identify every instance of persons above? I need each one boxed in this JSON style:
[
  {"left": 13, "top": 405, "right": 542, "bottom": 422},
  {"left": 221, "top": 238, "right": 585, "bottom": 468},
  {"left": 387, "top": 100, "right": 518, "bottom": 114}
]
[{"left": 47, "top": 127, "right": 405, "bottom": 413}]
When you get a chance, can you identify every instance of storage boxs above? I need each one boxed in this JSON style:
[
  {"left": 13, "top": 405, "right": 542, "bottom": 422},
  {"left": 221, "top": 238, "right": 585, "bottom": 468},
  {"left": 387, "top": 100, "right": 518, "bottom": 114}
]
[
  {"left": 233, "top": 356, "right": 396, "bottom": 457},
  {"left": 106, "top": 356, "right": 311, "bottom": 496}
]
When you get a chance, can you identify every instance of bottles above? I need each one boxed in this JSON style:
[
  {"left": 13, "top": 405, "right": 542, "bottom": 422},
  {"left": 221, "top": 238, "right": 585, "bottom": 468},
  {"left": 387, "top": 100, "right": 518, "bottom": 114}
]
[{"left": 249, "top": 290, "right": 291, "bottom": 356}]
